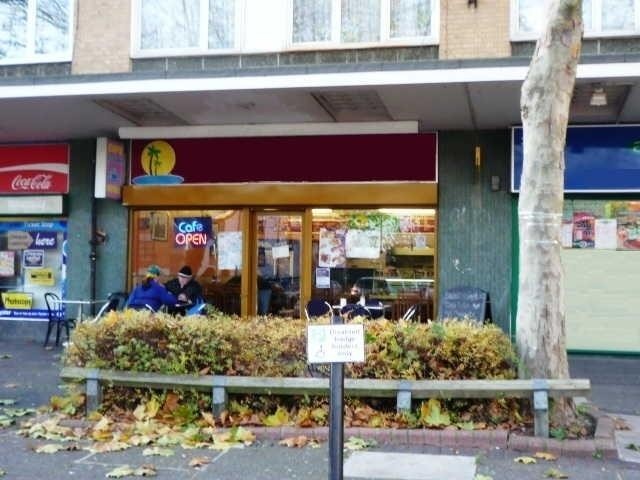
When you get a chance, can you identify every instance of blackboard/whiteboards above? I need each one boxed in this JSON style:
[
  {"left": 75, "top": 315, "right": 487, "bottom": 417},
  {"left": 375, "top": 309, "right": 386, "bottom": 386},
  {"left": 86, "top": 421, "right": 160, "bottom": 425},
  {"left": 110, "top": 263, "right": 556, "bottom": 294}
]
[{"left": 442, "top": 286, "right": 487, "bottom": 323}]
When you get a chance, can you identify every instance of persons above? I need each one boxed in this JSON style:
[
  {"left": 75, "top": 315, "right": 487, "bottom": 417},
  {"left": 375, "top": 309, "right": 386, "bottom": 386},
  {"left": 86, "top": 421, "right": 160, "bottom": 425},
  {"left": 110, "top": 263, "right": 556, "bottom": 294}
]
[
  {"left": 128, "top": 265, "right": 204, "bottom": 315},
  {"left": 198, "top": 267, "right": 234, "bottom": 290},
  {"left": 257, "top": 247, "right": 273, "bottom": 315},
  {"left": 319, "top": 226, "right": 347, "bottom": 268}
]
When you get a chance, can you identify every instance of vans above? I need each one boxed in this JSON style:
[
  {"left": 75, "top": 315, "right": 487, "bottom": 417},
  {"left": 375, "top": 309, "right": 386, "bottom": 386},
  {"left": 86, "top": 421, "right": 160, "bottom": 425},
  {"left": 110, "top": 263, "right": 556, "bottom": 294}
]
[{"left": 352, "top": 277, "right": 435, "bottom": 296}]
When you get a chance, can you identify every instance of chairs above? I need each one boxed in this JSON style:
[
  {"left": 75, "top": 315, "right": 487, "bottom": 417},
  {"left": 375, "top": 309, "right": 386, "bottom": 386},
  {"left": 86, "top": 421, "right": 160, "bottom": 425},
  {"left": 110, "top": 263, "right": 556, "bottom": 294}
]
[
  {"left": 44, "top": 291, "right": 74, "bottom": 345},
  {"left": 304, "top": 299, "right": 420, "bottom": 321}
]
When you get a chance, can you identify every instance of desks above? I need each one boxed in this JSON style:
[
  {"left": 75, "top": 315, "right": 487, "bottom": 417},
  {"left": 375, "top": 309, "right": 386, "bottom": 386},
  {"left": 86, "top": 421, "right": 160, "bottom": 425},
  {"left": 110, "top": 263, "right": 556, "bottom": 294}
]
[{"left": 53, "top": 291, "right": 195, "bottom": 324}]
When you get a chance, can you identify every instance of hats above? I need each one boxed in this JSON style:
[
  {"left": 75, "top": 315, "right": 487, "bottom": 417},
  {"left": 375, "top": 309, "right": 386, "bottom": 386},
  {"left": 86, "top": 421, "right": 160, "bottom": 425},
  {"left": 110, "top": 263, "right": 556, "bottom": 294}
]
[
  {"left": 146, "top": 264, "right": 161, "bottom": 278},
  {"left": 177, "top": 265, "right": 193, "bottom": 277}
]
